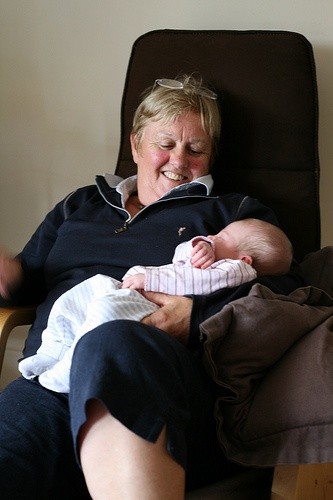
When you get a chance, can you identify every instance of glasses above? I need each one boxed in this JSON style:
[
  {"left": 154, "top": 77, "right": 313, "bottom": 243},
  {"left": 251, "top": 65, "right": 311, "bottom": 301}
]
[{"left": 153, "top": 78, "right": 218, "bottom": 108}]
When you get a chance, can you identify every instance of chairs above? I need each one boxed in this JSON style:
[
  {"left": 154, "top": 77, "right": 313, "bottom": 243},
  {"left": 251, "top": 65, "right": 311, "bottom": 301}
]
[{"left": 0, "top": 27, "right": 333, "bottom": 500}]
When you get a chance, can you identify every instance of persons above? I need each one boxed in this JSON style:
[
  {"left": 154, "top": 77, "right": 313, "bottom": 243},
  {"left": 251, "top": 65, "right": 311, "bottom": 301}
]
[
  {"left": 17, "top": 218, "right": 293, "bottom": 394},
  {"left": 0, "top": 70, "right": 303, "bottom": 500}
]
